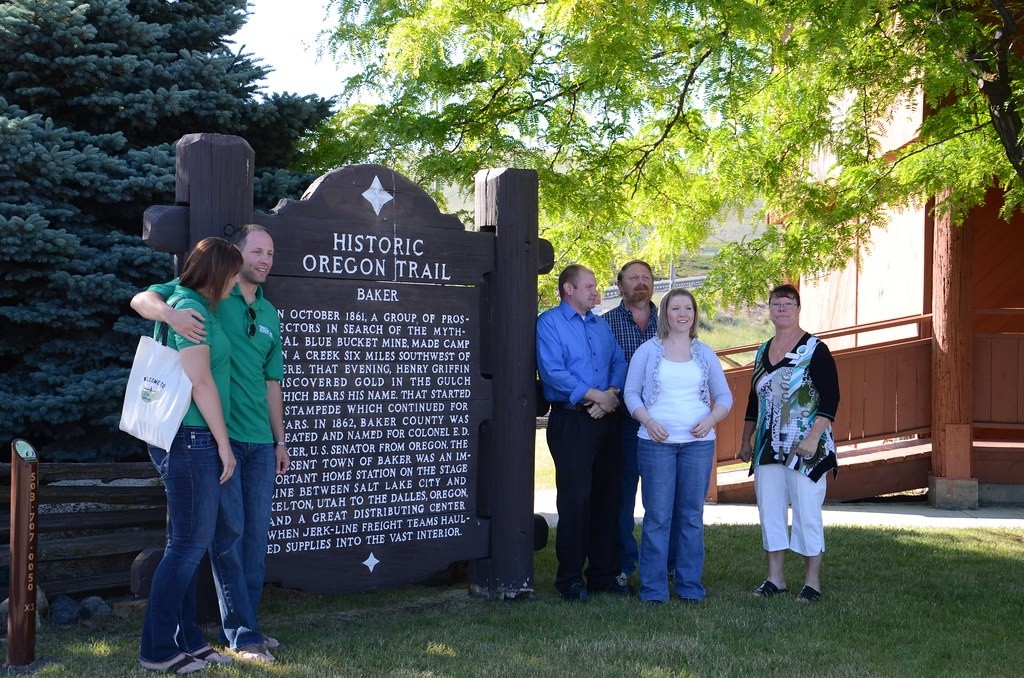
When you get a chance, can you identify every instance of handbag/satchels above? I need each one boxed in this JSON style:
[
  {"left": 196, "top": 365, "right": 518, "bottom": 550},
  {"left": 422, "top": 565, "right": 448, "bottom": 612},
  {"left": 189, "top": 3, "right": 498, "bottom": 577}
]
[{"left": 118, "top": 297, "right": 200, "bottom": 453}]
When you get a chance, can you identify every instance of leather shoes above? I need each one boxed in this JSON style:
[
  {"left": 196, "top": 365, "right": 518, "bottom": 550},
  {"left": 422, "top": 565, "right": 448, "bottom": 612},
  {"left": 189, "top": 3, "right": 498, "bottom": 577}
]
[
  {"left": 554, "top": 580, "right": 590, "bottom": 602},
  {"left": 586, "top": 579, "right": 635, "bottom": 595}
]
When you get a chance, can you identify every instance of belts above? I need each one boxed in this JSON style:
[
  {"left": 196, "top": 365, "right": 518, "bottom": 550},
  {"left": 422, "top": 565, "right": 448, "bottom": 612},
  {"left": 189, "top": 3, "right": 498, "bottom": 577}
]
[{"left": 551, "top": 401, "right": 587, "bottom": 412}]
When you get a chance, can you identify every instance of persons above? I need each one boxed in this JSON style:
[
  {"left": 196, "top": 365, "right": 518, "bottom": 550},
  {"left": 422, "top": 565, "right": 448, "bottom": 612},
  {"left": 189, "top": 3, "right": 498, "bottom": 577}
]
[
  {"left": 537, "top": 265, "right": 628, "bottom": 599},
  {"left": 623, "top": 288, "right": 733, "bottom": 605},
  {"left": 130, "top": 226, "right": 290, "bottom": 665},
  {"left": 739, "top": 284, "right": 840, "bottom": 604},
  {"left": 138, "top": 236, "right": 244, "bottom": 672},
  {"left": 599, "top": 260, "right": 659, "bottom": 588}
]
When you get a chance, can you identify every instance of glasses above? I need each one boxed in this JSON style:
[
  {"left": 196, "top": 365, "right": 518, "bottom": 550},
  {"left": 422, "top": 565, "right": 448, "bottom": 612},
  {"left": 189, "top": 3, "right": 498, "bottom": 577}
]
[
  {"left": 243, "top": 307, "right": 256, "bottom": 338},
  {"left": 770, "top": 301, "right": 797, "bottom": 308}
]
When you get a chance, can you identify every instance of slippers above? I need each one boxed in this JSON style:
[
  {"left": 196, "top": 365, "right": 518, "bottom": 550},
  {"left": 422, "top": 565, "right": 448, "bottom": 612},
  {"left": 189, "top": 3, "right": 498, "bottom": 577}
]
[
  {"left": 263, "top": 634, "right": 279, "bottom": 648},
  {"left": 797, "top": 585, "right": 821, "bottom": 603},
  {"left": 193, "top": 648, "right": 222, "bottom": 660},
  {"left": 147, "top": 654, "right": 201, "bottom": 675},
  {"left": 753, "top": 580, "right": 788, "bottom": 598},
  {"left": 224, "top": 644, "right": 276, "bottom": 662}
]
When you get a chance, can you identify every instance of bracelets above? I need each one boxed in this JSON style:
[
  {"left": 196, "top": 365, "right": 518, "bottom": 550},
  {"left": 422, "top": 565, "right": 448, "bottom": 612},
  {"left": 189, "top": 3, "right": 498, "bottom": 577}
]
[
  {"left": 274, "top": 441, "right": 287, "bottom": 446},
  {"left": 709, "top": 413, "right": 716, "bottom": 426}
]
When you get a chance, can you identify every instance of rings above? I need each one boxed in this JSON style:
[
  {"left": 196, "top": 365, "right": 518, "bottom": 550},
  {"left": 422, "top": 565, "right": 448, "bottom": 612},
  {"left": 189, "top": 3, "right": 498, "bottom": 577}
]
[
  {"left": 701, "top": 433, "right": 704, "bottom": 438},
  {"left": 805, "top": 454, "right": 809, "bottom": 457}
]
